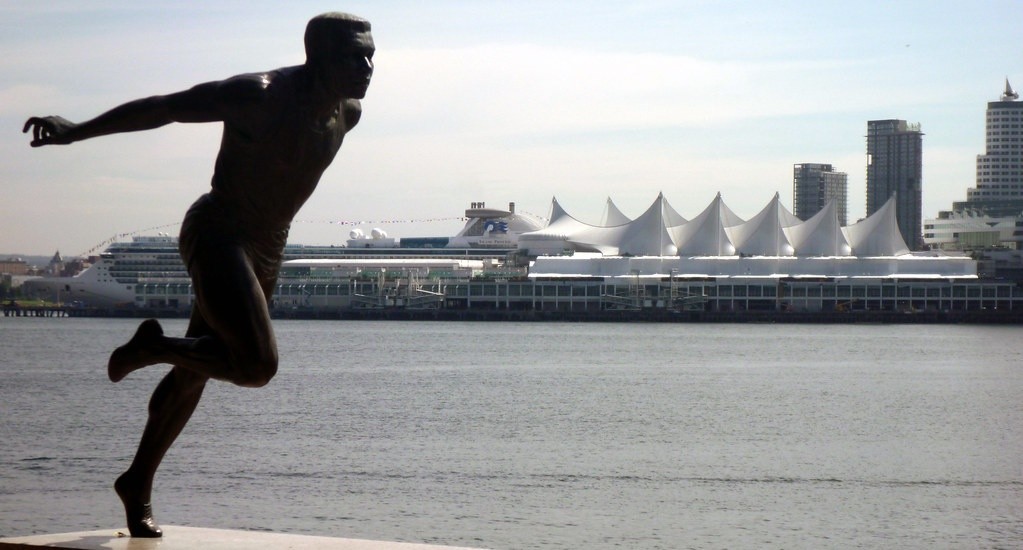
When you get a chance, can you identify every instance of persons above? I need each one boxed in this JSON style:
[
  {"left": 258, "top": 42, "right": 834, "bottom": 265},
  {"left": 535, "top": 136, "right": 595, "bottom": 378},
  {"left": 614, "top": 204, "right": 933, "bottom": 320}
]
[{"left": 22, "top": 12, "right": 375, "bottom": 538}]
